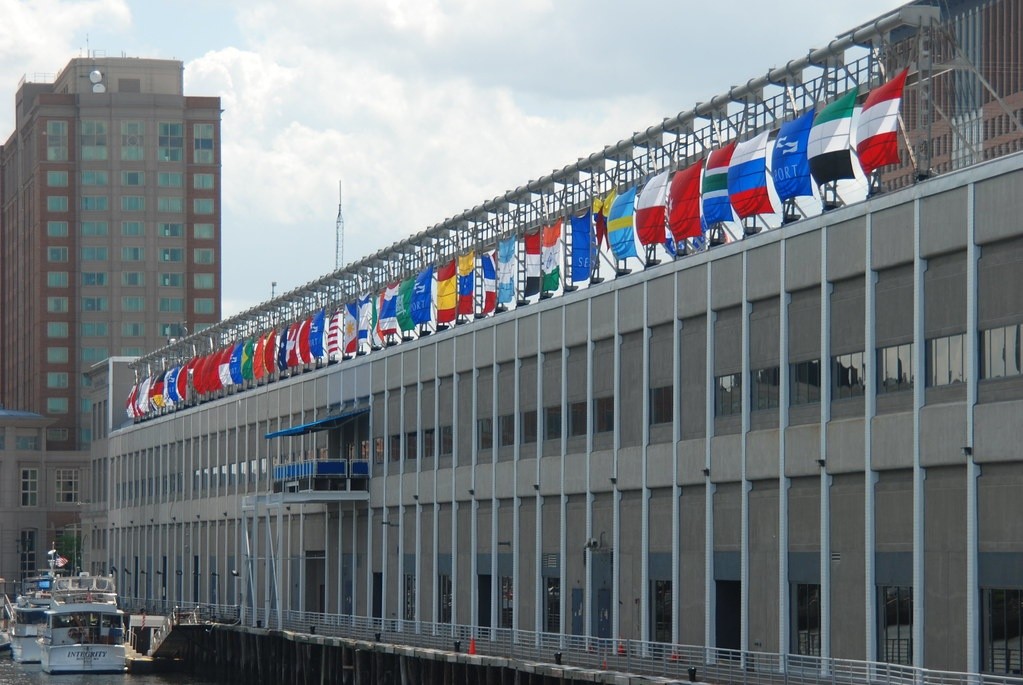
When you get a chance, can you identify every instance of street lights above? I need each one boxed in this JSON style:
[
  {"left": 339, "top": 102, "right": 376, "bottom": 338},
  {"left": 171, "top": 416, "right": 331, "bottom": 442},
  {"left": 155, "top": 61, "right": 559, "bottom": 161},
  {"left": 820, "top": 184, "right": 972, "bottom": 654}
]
[{"left": 64, "top": 523, "right": 82, "bottom": 575}]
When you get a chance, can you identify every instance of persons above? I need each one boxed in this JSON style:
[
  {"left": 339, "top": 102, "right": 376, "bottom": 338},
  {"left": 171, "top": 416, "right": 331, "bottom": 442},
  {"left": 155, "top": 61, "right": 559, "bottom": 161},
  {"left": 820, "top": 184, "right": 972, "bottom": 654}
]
[{"left": 71, "top": 615, "right": 83, "bottom": 627}]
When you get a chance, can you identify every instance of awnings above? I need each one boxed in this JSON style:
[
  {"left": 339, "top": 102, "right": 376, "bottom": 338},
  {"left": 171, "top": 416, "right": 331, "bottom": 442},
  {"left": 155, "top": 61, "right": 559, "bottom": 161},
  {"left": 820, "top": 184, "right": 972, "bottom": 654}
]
[{"left": 264, "top": 405, "right": 370, "bottom": 438}]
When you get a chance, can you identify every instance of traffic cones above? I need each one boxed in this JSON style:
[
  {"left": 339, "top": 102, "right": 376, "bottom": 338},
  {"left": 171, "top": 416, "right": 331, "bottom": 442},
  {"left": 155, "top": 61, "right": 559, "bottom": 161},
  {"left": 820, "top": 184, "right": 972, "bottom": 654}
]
[
  {"left": 468, "top": 636, "right": 477, "bottom": 654},
  {"left": 671, "top": 652, "right": 678, "bottom": 662},
  {"left": 618, "top": 642, "right": 624, "bottom": 655},
  {"left": 587, "top": 643, "right": 593, "bottom": 653},
  {"left": 603, "top": 659, "right": 607, "bottom": 670}
]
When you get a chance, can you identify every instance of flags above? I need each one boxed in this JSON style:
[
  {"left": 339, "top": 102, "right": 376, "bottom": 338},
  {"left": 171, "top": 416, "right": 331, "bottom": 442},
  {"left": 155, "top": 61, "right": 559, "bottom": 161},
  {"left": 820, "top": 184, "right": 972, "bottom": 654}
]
[
  {"left": 540, "top": 218, "right": 562, "bottom": 293},
  {"left": 856, "top": 66, "right": 908, "bottom": 174},
  {"left": 637, "top": 168, "right": 672, "bottom": 245},
  {"left": 607, "top": 184, "right": 653, "bottom": 260},
  {"left": 771, "top": 107, "right": 818, "bottom": 204},
  {"left": 481, "top": 248, "right": 498, "bottom": 315},
  {"left": 807, "top": 86, "right": 857, "bottom": 188},
  {"left": 571, "top": 207, "right": 603, "bottom": 282},
  {"left": 701, "top": 138, "right": 742, "bottom": 229},
  {"left": 727, "top": 129, "right": 782, "bottom": 220},
  {"left": 457, "top": 251, "right": 474, "bottom": 315},
  {"left": 125, "top": 260, "right": 457, "bottom": 419},
  {"left": 496, "top": 234, "right": 515, "bottom": 302},
  {"left": 668, "top": 159, "right": 702, "bottom": 243},
  {"left": 55, "top": 553, "right": 67, "bottom": 567},
  {"left": 523, "top": 232, "right": 541, "bottom": 298},
  {"left": 593, "top": 190, "right": 615, "bottom": 249}
]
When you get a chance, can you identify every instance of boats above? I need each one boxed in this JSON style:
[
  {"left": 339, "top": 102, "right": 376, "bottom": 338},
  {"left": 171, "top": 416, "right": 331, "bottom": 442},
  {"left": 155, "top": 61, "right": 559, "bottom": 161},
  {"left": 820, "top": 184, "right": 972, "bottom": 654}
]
[
  {"left": 38, "top": 571, "right": 127, "bottom": 674},
  {"left": 0, "top": 579, "right": 11, "bottom": 649},
  {"left": 4, "top": 589, "right": 51, "bottom": 664}
]
